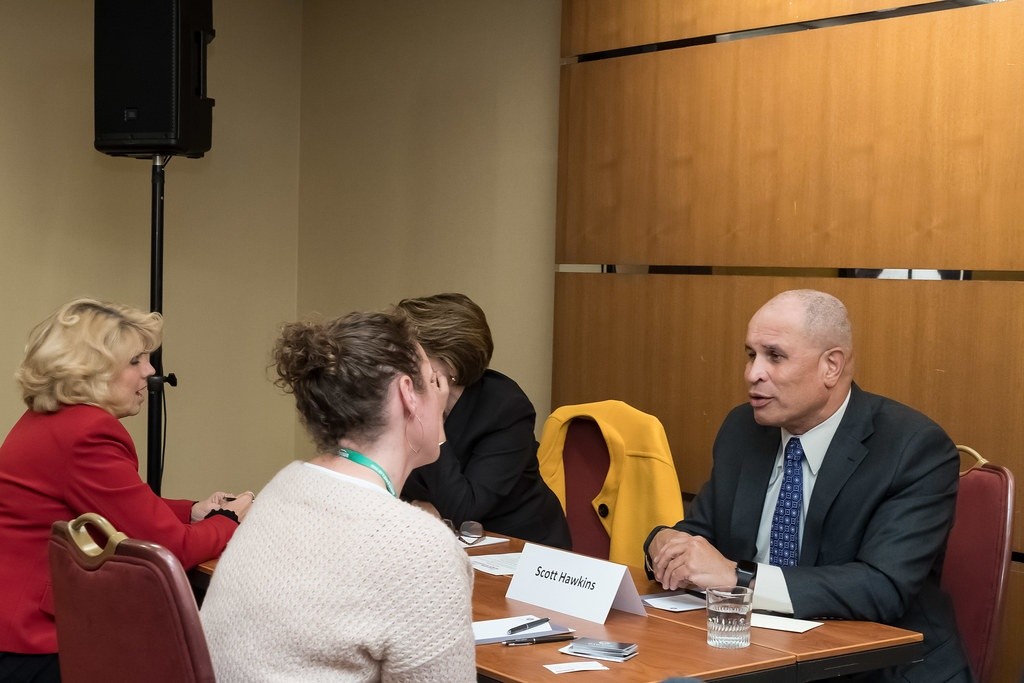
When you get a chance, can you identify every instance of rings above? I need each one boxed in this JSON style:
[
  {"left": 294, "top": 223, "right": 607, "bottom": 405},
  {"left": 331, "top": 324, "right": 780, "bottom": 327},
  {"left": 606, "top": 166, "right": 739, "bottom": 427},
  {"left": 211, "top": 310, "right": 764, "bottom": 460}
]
[{"left": 245, "top": 492, "right": 255, "bottom": 502}]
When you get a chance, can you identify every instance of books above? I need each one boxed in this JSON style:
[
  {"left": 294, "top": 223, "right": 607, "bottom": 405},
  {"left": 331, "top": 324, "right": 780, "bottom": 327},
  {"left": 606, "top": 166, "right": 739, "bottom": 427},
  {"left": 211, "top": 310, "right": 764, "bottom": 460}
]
[{"left": 472, "top": 615, "right": 576, "bottom": 646}]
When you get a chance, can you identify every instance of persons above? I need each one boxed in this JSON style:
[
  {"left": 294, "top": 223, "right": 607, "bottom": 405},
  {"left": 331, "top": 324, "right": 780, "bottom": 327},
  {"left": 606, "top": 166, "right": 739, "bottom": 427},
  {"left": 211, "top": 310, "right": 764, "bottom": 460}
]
[
  {"left": 0, "top": 296, "right": 253, "bottom": 683},
  {"left": 199, "top": 304, "right": 478, "bottom": 683},
  {"left": 643, "top": 288, "right": 972, "bottom": 683},
  {"left": 398, "top": 293, "right": 573, "bottom": 552}
]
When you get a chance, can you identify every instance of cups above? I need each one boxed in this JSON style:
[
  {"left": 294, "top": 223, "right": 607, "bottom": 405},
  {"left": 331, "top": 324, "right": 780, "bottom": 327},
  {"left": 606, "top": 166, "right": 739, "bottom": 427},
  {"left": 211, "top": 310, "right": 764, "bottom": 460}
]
[{"left": 706, "top": 586, "right": 754, "bottom": 648}]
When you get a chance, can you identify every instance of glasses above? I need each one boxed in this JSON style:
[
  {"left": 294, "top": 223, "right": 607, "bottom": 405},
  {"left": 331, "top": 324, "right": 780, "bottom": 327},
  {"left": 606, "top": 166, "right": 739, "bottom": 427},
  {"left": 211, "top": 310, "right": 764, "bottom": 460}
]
[{"left": 443, "top": 519, "right": 485, "bottom": 544}]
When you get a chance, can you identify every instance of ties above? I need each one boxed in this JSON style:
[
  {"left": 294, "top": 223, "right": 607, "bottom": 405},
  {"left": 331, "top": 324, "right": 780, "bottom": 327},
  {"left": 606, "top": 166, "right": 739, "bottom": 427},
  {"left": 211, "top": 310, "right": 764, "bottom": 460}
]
[{"left": 768, "top": 437, "right": 805, "bottom": 566}]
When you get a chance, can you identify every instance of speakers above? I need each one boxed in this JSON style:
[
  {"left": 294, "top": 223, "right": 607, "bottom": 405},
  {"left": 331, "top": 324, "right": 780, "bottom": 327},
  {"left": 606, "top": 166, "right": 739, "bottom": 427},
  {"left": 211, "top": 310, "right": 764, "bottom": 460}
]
[{"left": 93, "top": 0, "right": 216, "bottom": 160}]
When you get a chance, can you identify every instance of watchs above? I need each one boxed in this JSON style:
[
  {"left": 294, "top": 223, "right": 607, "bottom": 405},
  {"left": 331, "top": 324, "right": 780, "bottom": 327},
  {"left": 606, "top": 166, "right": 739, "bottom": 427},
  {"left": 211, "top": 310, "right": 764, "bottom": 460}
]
[{"left": 731, "top": 560, "right": 758, "bottom": 595}]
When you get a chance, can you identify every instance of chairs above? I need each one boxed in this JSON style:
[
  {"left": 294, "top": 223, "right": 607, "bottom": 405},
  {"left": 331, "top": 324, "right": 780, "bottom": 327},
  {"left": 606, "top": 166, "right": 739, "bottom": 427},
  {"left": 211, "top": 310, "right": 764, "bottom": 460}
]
[
  {"left": 48, "top": 513, "right": 217, "bottom": 683},
  {"left": 944, "top": 446, "right": 1014, "bottom": 683},
  {"left": 538, "top": 402, "right": 684, "bottom": 569}
]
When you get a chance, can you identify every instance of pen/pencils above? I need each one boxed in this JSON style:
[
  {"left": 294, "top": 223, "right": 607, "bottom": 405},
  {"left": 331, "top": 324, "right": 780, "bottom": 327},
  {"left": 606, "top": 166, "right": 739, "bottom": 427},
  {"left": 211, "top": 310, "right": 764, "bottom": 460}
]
[
  {"left": 507, "top": 617, "right": 551, "bottom": 635},
  {"left": 222, "top": 497, "right": 237, "bottom": 501},
  {"left": 501, "top": 635, "right": 578, "bottom": 647},
  {"left": 682, "top": 588, "right": 706, "bottom": 600}
]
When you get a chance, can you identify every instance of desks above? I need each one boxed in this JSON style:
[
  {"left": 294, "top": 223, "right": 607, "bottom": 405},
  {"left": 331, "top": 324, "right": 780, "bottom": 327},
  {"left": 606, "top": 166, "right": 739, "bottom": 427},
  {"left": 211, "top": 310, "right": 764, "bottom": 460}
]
[{"left": 193, "top": 526, "right": 925, "bottom": 683}]
